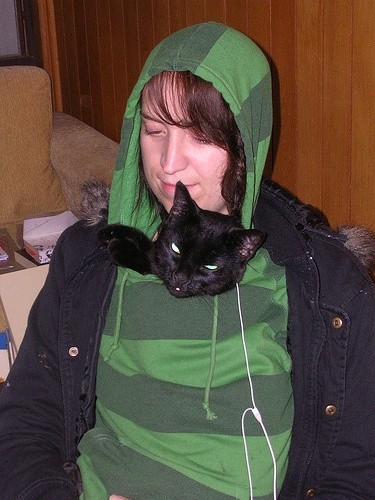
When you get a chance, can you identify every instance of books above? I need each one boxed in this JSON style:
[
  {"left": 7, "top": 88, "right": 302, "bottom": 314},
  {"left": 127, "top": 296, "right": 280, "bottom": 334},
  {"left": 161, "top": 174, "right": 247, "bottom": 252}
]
[{"left": 13, "top": 209, "right": 79, "bottom": 270}]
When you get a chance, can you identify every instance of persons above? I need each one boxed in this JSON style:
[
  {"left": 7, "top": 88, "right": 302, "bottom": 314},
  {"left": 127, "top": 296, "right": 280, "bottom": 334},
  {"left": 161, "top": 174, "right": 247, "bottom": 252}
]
[{"left": 0, "top": 21, "right": 375, "bottom": 499}]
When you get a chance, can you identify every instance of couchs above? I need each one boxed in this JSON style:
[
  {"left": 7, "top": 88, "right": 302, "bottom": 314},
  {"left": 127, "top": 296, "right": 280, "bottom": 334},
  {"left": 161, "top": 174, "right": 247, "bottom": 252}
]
[{"left": 0, "top": 53, "right": 119, "bottom": 336}]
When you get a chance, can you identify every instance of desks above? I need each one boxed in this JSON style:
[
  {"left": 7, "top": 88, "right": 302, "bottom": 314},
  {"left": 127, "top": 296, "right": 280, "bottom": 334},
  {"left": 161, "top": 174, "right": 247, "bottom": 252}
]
[{"left": 0, "top": 264, "right": 50, "bottom": 369}]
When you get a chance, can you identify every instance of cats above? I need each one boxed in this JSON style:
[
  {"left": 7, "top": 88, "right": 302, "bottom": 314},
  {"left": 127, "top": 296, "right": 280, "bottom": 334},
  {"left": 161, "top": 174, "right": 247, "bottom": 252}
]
[{"left": 97, "top": 180, "right": 266, "bottom": 322}]
